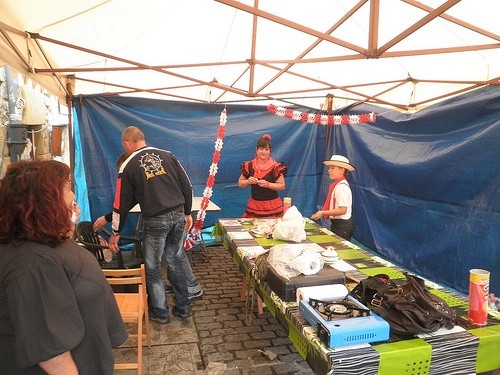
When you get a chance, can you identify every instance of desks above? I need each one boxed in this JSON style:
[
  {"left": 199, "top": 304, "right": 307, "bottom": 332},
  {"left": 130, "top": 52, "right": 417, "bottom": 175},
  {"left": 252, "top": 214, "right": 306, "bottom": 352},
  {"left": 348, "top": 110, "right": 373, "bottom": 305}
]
[
  {"left": 127, "top": 197, "right": 221, "bottom": 258},
  {"left": 211, "top": 216, "right": 500, "bottom": 375}
]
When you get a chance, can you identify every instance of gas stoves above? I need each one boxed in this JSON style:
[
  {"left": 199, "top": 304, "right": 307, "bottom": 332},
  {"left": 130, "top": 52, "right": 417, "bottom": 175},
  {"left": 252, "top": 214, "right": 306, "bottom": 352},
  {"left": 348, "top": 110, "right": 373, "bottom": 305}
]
[{"left": 299, "top": 294, "right": 390, "bottom": 349}]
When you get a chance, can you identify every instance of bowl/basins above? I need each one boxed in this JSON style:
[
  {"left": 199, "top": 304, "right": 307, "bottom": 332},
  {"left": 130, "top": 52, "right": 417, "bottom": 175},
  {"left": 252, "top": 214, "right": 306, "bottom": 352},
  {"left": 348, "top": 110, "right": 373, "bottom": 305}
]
[{"left": 320, "top": 251, "right": 339, "bottom": 264}]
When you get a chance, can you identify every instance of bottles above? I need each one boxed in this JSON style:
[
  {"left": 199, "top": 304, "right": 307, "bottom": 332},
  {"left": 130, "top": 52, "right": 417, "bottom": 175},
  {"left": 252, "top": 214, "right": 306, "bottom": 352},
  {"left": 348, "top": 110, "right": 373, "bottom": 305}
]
[{"left": 489, "top": 298, "right": 497, "bottom": 310}]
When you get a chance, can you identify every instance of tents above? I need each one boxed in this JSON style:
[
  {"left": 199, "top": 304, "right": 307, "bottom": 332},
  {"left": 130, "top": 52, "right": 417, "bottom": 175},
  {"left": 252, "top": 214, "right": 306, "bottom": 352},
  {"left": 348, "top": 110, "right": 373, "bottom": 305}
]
[{"left": 0, "top": 0, "right": 500, "bottom": 300}]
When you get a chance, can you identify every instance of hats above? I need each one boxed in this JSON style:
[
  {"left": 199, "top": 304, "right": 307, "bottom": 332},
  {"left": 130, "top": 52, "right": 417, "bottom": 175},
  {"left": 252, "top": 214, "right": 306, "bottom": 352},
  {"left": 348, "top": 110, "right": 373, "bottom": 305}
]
[{"left": 323, "top": 154, "right": 357, "bottom": 172}]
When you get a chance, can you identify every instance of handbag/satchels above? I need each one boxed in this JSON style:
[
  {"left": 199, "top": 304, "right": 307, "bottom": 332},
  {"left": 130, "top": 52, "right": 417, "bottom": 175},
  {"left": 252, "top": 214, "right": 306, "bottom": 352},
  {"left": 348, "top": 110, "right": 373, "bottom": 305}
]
[{"left": 352, "top": 272, "right": 454, "bottom": 336}]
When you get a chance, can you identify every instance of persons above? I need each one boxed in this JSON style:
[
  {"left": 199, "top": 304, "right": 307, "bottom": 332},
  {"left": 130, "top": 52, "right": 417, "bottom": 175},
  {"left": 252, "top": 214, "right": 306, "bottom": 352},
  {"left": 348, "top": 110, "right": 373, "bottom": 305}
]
[
  {"left": 108, "top": 126, "right": 192, "bottom": 325},
  {"left": 311, "top": 155, "right": 356, "bottom": 242},
  {"left": 93, "top": 152, "right": 203, "bottom": 302},
  {"left": 237, "top": 134, "right": 288, "bottom": 219},
  {"left": 0, "top": 161, "right": 128, "bottom": 375}
]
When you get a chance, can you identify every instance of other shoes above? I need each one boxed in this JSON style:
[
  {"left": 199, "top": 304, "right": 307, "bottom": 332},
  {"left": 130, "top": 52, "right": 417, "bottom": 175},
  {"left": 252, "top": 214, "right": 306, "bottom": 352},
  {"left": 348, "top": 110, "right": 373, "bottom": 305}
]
[
  {"left": 172, "top": 306, "right": 193, "bottom": 318},
  {"left": 188, "top": 290, "right": 203, "bottom": 300},
  {"left": 151, "top": 313, "right": 170, "bottom": 324},
  {"left": 164, "top": 284, "right": 173, "bottom": 293}
]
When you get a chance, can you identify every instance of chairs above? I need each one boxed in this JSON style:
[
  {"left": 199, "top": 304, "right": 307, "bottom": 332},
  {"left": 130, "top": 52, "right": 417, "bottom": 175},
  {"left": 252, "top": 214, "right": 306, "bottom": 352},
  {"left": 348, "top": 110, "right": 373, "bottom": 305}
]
[
  {"left": 75, "top": 221, "right": 141, "bottom": 292},
  {"left": 98, "top": 264, "right": 152, "bottom": 375}
]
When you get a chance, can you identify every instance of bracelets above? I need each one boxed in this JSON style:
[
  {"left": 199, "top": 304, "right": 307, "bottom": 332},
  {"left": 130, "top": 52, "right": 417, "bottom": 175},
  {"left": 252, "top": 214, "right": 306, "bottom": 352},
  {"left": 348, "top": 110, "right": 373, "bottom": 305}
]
[
  {"left": 112, "top": 229, "right": 120, "bottom": 236},
  {"left": 266, "top": 181, "right": 269, "bottom": 189}
]
